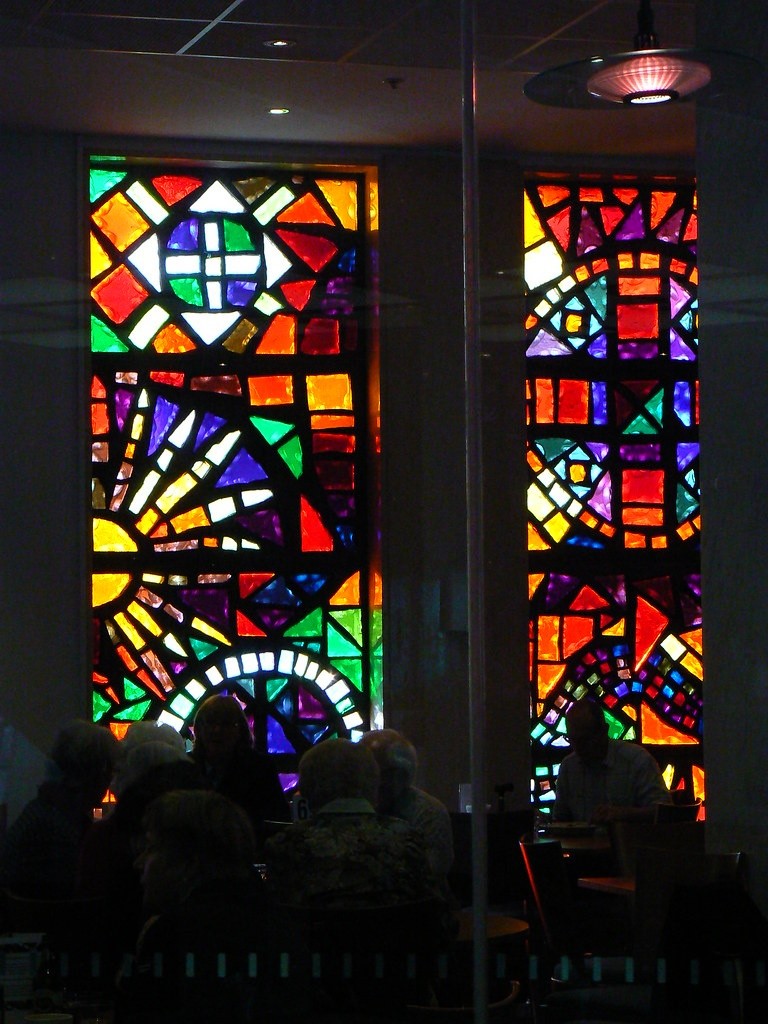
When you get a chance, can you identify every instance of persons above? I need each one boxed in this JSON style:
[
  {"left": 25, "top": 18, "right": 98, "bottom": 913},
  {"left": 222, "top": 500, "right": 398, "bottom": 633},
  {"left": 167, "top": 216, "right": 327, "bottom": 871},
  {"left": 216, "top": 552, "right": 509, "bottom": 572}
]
[
  {"left": 359, "top": 727, "right": 456, "bottom": 891},
  {"left": 115, "top": 791, "right": 321, "bottom": 1024},
  {"left": 272, "top": 738, "right": 436, "bottom": 909},
  {"left": 546, "top": 698, "right": 672, "bottom": 987},
  {"left": 184, "top": 695, "right": 294, "bottom": 843},
  {"left": 75, "top": 740, "right": 197, "bottom": 893},
  {"left": 0, "top": 719, "right": 114, "bottom": 902}
]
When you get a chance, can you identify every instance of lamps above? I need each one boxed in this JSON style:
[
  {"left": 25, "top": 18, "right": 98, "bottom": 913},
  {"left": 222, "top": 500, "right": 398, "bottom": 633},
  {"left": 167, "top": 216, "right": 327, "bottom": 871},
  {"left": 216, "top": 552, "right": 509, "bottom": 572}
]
[{"left": 522, "top": 0, "right": 768, "bottom": 109}]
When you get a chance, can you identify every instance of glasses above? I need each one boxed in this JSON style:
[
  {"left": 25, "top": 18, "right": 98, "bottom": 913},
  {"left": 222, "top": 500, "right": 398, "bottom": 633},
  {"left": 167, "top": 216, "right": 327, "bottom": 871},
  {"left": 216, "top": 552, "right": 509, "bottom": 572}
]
[{"left": 565, "top": 725, "right": 602, "bottom": 744}]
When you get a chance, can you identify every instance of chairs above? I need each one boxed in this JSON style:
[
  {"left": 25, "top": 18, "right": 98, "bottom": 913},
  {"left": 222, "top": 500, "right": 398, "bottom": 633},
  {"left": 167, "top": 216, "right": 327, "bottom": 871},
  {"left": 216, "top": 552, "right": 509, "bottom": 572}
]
[
  {"left": 518, "top": 840, "right": 579, "bottom": 996},
  {"left": 0, "top": 884, "right": 110, "bottom": 984},
  {"left": 448, "top": 807, "right": 535, "bottom": 910},
  {"left": 605, "top": 818, "right": 705, "bottom": 877},
  {"left": 269, "top": 894, "right": 446, "bottom": 1024},
  {"left": 632, "top": 848, "right": 752, "bottom": 971}
]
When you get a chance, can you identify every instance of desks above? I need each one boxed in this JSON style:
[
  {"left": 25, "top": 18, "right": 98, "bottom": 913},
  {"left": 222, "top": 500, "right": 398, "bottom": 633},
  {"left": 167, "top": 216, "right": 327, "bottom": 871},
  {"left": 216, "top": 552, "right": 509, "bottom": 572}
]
[
  {"left": 578, "top": 873, "right": 635, "bottom": 897},
  {"left": 453, "top": 909, "right": 528, "bottom": 944},
  {"left": 542, "top": 833, "right": 611, "bottom": 854}
]
